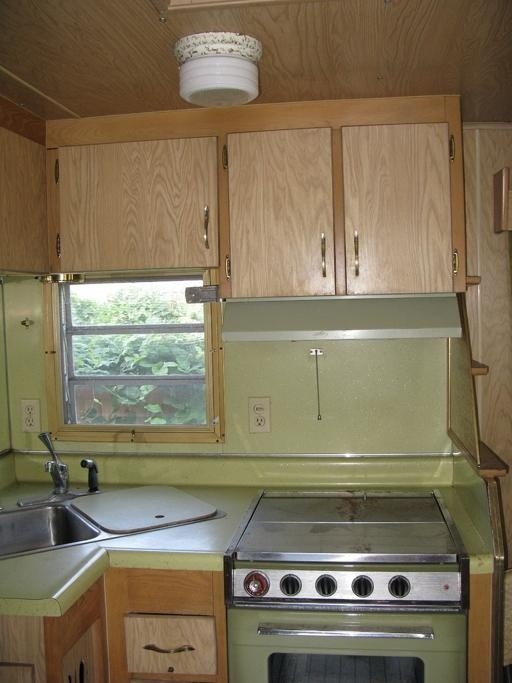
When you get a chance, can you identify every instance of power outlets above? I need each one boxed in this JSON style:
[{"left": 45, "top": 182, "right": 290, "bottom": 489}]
[
  {"left": 22, "top": 398, "right": 41, "bottom": 433},
  {"left": 249, "top": 397, "right": 271, "bottom": 433}
]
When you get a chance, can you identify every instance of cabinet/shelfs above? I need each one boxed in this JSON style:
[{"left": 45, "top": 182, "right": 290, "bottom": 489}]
[
  {"left": 219, "top": 94, "right": 466, "bottom": 300},
  {"left": 0, "top": 566, "right": 228, "bottom": 683},
  {"left": 43, "top": 108, "right": 220, "bottom": 275}
]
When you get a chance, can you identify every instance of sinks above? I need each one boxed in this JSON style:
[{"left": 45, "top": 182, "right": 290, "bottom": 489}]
[{"left": 0, "top": 503, "right": 101, "bottom": 562}]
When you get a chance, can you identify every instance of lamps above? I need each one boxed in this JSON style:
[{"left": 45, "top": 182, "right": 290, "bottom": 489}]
[{"left": 174, "top": 31, "right": 264, "bottom": 110}]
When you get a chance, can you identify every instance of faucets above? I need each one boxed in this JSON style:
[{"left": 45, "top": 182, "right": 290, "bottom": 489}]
[
  {"left": 80, "top": 457, "right": 100, "bottom": 493},
  {"left": 37, "top": 432, "right": 68, "bottom": 495}
]
